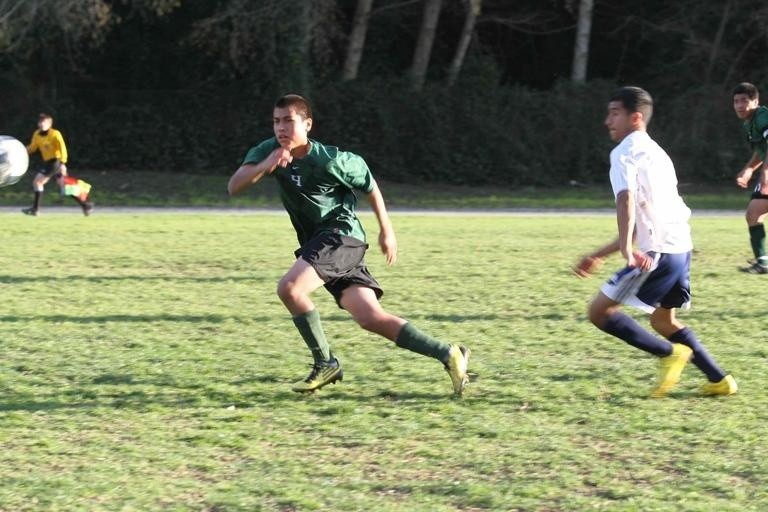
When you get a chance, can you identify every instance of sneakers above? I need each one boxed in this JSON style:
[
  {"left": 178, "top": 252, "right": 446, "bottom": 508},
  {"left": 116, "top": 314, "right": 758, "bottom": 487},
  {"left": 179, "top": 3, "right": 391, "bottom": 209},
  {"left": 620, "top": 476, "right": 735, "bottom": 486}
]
[
  {"left": 83, "top": 200, "right": 93, "bottom": 216},
  {"left": 443, "top": 342, "right": 471, "bottom": 396},
  {"left": 649, "top": 344, "right": 694, "bottom": 397},
  {"left": 735, "top": 258, "right": 768, "bottom": 275},
  {"left": 690, "top": 373, "right": 738, "bottom": 397},
  {"left": 21, "top": 207, "right": 38, "bottom": 217},
  {"left": 291, "top": 357, "right": 344, "bottom": 392}
]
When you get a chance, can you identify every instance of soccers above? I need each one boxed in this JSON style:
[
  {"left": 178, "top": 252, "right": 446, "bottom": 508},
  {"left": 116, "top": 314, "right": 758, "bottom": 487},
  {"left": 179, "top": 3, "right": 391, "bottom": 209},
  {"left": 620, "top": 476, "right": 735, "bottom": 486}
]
[{"left": 0, "top": 134, "right": 30, "bottom": 188}]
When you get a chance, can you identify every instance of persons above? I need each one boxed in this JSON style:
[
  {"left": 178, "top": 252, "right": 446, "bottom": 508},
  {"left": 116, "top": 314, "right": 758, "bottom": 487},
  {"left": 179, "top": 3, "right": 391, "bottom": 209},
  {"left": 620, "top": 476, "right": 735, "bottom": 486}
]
[
  {"left": 228, "top": 93, "right": 470, "bottom": 398},
  {"left": 571, "top": 86, "right": 738, "bottom": 397},
  {"left": 21, "top": 112, "right": 93, "bottom": 217},
  {"left": 732, "top": 83, "right": 768, "bottom": 275}
]
